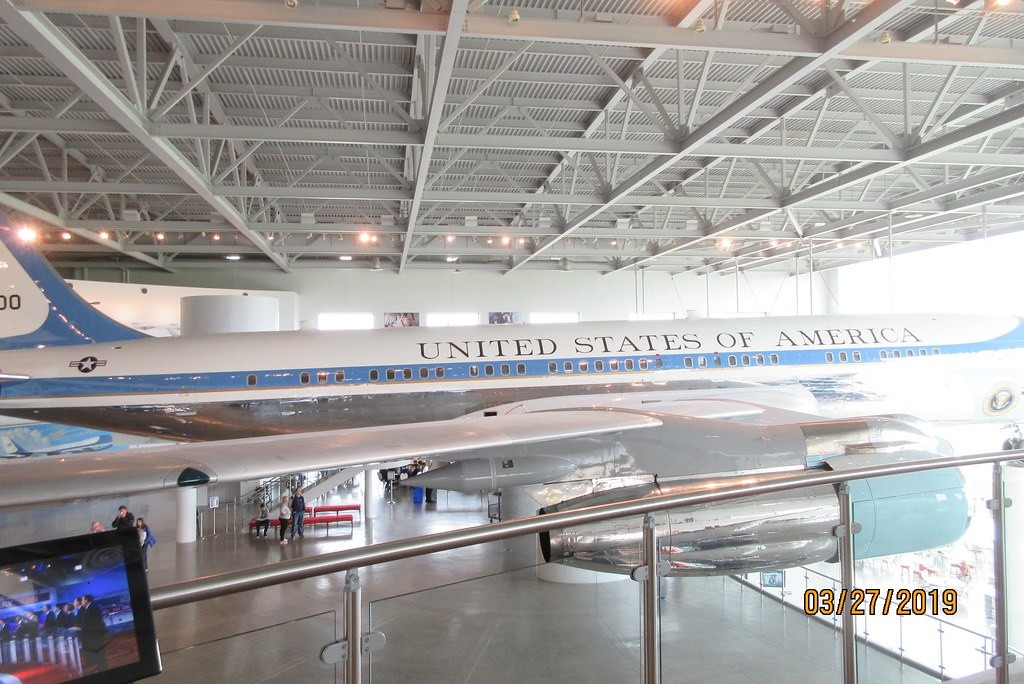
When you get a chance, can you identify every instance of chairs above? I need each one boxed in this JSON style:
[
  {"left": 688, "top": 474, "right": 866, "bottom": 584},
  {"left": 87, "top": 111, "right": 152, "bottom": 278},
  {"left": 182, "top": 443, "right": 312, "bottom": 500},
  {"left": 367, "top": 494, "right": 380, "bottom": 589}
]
[{"left": 376, "top": 471, "right": 423, "bottom": 487}]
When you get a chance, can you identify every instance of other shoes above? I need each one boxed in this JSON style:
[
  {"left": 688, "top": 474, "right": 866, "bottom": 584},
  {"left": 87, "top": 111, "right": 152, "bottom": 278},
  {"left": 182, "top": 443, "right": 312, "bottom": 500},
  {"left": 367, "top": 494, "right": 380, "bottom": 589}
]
[
  {"left": 256, "top": 536, "right": 259, "bottom": 538},
  {"left": 264, "top": 536, "right": 267, "bottom": 538},
  {"left": 280, "top": 539, "right": 288, "bottom": 545}
]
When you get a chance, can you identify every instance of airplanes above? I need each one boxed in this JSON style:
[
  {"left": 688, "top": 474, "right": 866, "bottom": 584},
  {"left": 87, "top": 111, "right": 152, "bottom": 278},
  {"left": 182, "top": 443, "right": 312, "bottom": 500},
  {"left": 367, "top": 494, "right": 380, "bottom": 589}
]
[{"left": 1, "top": 226, "right": 1023, "bottom": 583}]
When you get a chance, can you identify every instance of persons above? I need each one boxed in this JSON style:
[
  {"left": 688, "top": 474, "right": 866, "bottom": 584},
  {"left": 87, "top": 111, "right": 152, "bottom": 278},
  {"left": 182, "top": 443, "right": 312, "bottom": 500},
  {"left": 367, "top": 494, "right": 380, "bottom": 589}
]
[
  {"left": 111, "top": 505, "right": 135, "bottom": 529},
  {"left": 1, "top": 593, "right": 110, "bottom": 675},
  {"left": 255, "top": 503, "right": 270, "bottom": 538},
  {"left": 134, "top": 518, "right": 151, "bottom": 572},
  {"left": 247, "top": 472, "right": 307, "bottom": 508},
  {"left": 87, "top": 520, "right": 106, "bottom": 535},
  {"left": 377, "top": 459, "right": 434, "bottom": 504},
  {"left": 278, "top": 495, "right": 291, "bottom": 544},
  {"left": 291, "top": 486, "right": 307, "bottom": 540}
]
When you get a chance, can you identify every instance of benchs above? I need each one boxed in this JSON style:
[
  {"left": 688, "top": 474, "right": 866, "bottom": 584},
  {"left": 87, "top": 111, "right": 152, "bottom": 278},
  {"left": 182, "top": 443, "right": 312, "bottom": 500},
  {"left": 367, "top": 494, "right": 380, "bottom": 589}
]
[
  {"left": 249, "top": 514, "right": 353, "bottom": 540},
  {"left": 314, "top": 504, "right": 361, "bottom": 523},
  {"left": 305, "top": 507, "right": 313, "bottom": 516}
]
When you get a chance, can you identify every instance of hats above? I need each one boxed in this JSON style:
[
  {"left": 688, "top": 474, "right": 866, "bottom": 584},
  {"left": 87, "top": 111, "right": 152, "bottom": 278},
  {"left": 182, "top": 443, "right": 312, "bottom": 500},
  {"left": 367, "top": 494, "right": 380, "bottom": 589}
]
[{"left": 260, "top": 503, "right": 266, "bottom": 507}]
